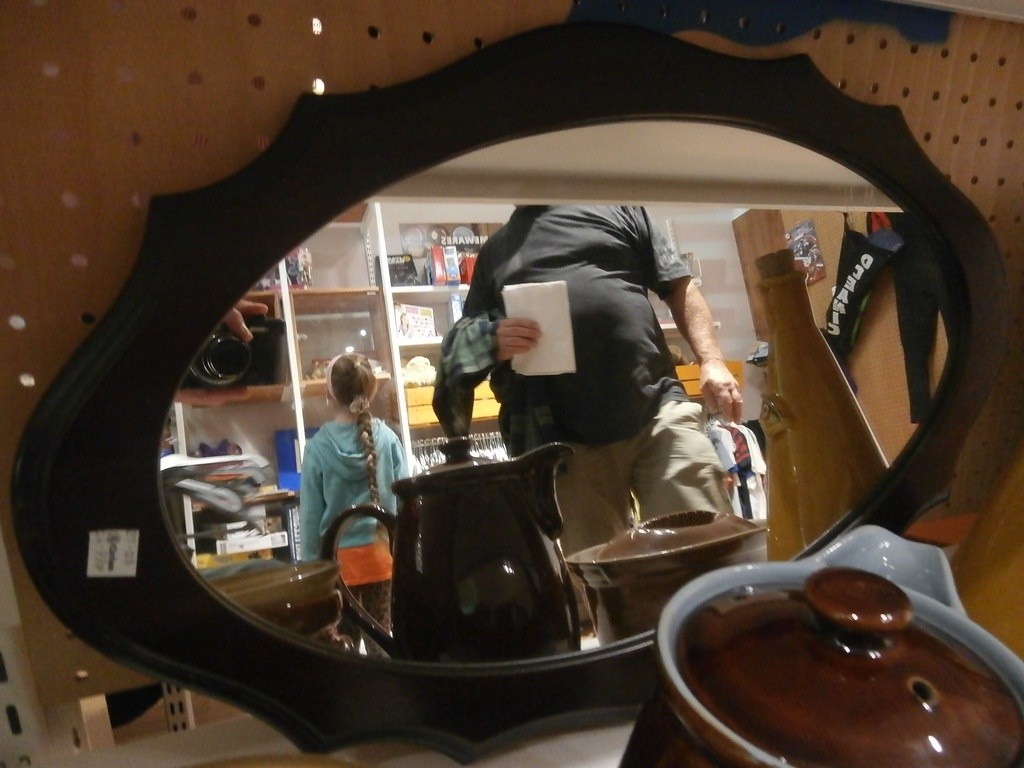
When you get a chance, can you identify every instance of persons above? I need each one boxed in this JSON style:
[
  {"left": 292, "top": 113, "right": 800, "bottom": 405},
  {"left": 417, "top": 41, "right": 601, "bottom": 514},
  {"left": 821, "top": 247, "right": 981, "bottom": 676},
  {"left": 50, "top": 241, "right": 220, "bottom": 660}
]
[
  {"left": 299, "top": 354, "right": 412, "bottom": 658},
  {"left": 2, "top": 300, "right": 269, "bottom": 734},
  {"left": 463, "top": 203, "right": 745, "bottom": 646}
]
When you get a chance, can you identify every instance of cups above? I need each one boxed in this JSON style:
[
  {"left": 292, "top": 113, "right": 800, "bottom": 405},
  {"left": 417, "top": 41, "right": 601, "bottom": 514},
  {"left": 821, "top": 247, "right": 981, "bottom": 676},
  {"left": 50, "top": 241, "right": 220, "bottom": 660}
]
[
  {"left": 411, "top": 430, "right": 516, "bottom": 469},
  {"left": 564, "top": 512, "right": 767, "bottom": 648}
]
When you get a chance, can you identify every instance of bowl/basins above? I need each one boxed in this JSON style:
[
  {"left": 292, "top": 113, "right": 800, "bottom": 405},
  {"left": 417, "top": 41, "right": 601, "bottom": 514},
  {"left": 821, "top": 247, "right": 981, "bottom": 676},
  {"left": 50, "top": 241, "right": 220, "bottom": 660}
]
[
  {"left": 260, "top": 589, "right": 344, "bottom": 639},
  {"left": 195, "top": 559, "right": 342, "bottom": 614}
]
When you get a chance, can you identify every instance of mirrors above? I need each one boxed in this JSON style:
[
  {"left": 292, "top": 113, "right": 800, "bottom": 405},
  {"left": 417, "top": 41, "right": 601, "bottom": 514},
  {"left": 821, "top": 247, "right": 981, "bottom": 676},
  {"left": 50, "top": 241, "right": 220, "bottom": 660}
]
[{"left": 11, "top": 21, "right": 1011, "bottom": 764}]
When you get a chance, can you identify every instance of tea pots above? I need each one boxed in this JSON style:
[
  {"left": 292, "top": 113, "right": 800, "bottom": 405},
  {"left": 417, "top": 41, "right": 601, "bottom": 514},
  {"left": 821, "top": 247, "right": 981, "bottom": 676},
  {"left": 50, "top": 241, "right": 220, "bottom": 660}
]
[
  {"left": 656, "top": 524, "right": 1024, "bottom": 768},
  {"left": 320, "top": 439, "right": 578, "bottom": 663}
]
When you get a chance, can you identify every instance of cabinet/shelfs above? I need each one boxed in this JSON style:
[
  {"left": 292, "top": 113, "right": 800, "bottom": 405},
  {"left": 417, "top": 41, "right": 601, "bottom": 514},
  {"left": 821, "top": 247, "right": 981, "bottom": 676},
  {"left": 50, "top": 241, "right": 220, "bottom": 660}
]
[{"left": 161, "top": 201, "right": 723, "bottom": 562}]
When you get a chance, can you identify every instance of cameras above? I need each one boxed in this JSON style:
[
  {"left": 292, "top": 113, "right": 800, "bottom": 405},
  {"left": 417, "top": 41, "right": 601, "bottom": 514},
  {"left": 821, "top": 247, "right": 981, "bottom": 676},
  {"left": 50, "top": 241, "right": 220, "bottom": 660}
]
[{"left": 181, "top": 316, "right": 289, "bottom": 390}]
